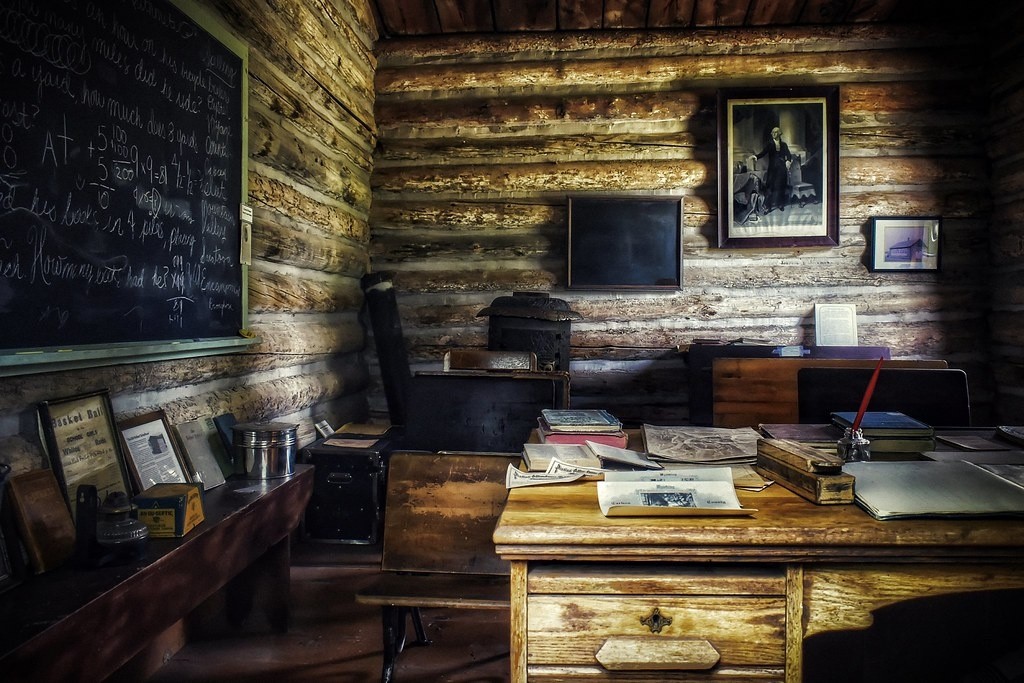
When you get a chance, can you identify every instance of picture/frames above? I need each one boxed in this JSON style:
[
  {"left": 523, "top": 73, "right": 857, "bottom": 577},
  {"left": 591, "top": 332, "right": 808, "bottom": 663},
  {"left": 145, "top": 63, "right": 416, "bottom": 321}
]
[
  {"left": 869, "top": 216, "right": 941, "bottom": 274},
  {"left": 713, "top": 83, "right": 840, "bottom": 247},
  {"left": 117, "top": 410, "right": 193, "bottom": 493},
  {"left": 42, "top": 387, "right": 135, "bottom": 525}
]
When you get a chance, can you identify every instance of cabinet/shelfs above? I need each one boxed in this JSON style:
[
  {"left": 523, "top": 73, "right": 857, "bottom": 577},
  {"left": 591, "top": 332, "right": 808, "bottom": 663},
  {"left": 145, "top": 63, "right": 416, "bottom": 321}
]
[{"left": 0, "top": 464, "right": 321, "bottom": 683}]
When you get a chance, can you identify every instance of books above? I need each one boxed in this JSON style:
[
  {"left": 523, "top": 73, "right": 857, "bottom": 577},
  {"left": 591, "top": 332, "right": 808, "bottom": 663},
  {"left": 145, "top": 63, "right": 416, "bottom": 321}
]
[
  {"left": 520, "top": 409, "right": 665, "bottom": 472},
  {"left": 172, "top": 411, "right": 238, "bottom": 490},
  {"left": 5, "top": 468, "right": 77, "bottom": 575},
  {"left": 757, "top": 410, "right": 937, "bottom": 455}
]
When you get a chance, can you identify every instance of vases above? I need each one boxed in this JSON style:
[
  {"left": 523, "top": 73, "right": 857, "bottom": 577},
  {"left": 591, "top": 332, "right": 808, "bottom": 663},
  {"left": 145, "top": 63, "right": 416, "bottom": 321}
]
[{"left": 563, "top": 194, "right": 684, "bottom": 292}]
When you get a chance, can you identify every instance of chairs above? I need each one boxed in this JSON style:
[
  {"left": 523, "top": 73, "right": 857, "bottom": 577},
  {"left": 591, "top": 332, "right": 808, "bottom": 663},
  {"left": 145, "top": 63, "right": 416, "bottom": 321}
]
[
  {"left": 489, "top": 443, "right": 1024, "bottom": 683},
  {"left": 354, "top": 371, "right": 572, "bottom": 683},
  {"left": 798, "top": 366, "right": 971, "bottom": 432}
]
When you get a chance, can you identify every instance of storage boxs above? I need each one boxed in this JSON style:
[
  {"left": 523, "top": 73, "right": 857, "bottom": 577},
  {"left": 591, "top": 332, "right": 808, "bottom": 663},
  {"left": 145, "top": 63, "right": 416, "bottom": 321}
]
[{"left": 132, "top": 481, "right": 206, "bottom": 540}]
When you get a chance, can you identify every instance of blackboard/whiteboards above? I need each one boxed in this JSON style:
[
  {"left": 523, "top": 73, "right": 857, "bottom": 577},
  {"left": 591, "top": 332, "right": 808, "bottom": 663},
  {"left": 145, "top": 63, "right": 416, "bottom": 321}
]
[{"left": 1, "top": 0, "right": 262, "bottom": 375}]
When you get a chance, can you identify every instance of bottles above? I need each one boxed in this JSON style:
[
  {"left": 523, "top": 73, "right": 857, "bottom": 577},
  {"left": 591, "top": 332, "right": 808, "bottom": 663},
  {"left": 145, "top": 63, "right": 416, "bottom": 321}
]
[{"left": 836, "top": 428, "right": 871, "bottom": 462}]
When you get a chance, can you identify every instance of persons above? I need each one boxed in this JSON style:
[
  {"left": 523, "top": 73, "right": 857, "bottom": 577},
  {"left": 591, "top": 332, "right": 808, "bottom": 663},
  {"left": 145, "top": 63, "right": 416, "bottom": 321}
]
[{"left": 748, "top": 127, "right": 792, "bottom": 215}]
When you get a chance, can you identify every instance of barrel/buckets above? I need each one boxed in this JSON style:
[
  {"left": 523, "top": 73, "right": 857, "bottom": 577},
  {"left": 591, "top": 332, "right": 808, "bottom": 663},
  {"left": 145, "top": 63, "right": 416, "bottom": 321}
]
[{"left": 226, "top": 419, "right": 299, "bottom": 481}]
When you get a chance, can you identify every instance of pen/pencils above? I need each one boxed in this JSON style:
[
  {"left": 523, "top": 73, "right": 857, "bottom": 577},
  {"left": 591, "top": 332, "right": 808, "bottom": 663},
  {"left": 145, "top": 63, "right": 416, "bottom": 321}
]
[{"left": 843, "top": 356, "right": 884, "bottom": 456}]
